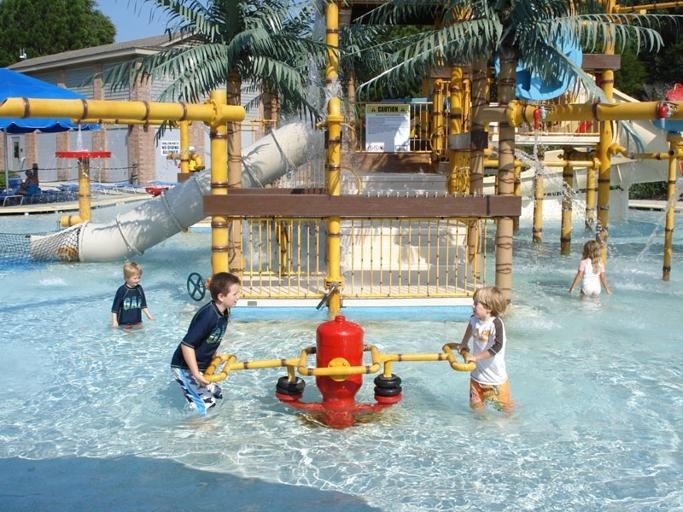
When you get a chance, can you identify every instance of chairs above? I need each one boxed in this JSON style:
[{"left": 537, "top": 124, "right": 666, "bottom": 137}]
[{"left": 1, "top": 172, "right": 181, "bottom": 206}]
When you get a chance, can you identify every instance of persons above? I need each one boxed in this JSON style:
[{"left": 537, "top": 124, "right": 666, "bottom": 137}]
[
  {"left": 15, "top": 168, "right": 38, "bottom": 199},
  {"left": 170, "top": 271, "right": 242, "bottom": 427},
  {"left": 455, "top": 285, "right": 518, "bottom": 420},
  {"left": 110, "top": 260, "right": 153, "bottom": 332},
  {"left": 565, "top": 237, "right": 614, "bottom": 301}
]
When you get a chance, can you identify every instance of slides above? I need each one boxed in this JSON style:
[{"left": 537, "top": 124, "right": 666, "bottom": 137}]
[{"left": 28, "top": 119, "right": 326, "bottom": 262}]
